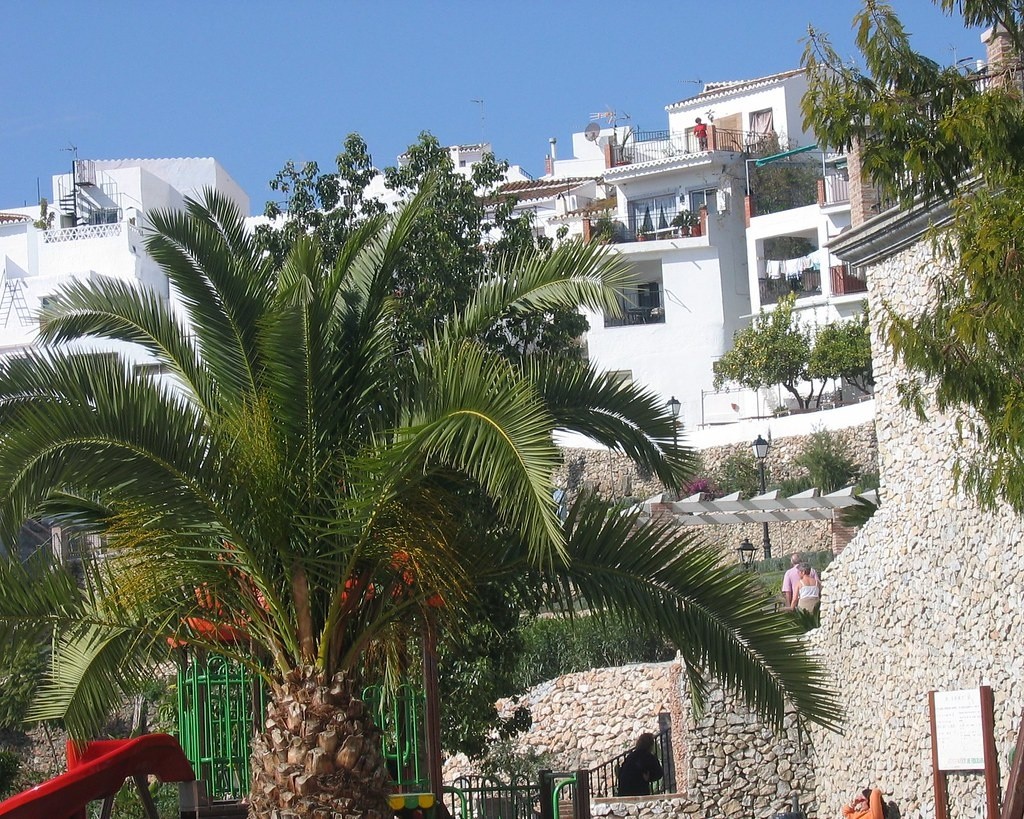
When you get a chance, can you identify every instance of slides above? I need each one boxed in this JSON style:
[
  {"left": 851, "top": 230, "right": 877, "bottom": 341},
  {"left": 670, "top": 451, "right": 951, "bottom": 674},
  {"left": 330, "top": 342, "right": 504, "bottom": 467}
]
[{"left": 0, "top": 731, "right": 190, "bottom": 818}]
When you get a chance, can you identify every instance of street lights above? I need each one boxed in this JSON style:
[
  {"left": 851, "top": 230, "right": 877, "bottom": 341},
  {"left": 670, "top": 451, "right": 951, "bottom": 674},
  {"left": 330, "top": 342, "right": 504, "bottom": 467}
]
[
  {"left": 736, "top": 538, "right": 758, "bottom": 577},
  {"left": 752, "top": 433, "right": 773, "bottom": 559},
  {"left": 665, "top": 398, "right": 683, "bottom": 502}
]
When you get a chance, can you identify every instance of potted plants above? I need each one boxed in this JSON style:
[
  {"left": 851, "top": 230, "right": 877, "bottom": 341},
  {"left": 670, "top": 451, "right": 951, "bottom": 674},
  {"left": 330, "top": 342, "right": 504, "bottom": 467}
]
[
  {"left": 591, "top": 203, "right": 623, "bottom": 245},
  {"left": 670, "top": 209, "right": 698, "bottom": 237},
  {"left": 688, "top": 211, "right": 701, "bottom": 236},
  {"left": 636, "top": 226, "right": 647, "bottom": 241},
  {"left": 616, "top": 126, "right": 635, "bottom": 166}
]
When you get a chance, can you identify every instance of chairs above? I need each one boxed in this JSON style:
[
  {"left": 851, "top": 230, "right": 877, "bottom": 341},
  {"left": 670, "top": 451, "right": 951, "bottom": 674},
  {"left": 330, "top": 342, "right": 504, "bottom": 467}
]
[{"left": 622, "top": 304, "right": 651, "bottom": 325}]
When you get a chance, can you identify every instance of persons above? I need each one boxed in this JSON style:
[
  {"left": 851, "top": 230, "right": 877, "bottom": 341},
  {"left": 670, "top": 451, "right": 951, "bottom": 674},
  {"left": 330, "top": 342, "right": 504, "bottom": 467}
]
[
  {"left": 693, "top": 117, "right": 707, "bottom": 151},
  {"left": 841, "top": 787, "right": 888, "bottom": 819},
  {"left": 781, "top": 554, "right": 819, "bottom": 607},
  {"left": 789, "top": 563, "right": 821, "bottom": 613},
  {"left": 616, "top": 733, "right": 663, "bottom": 797}
]
